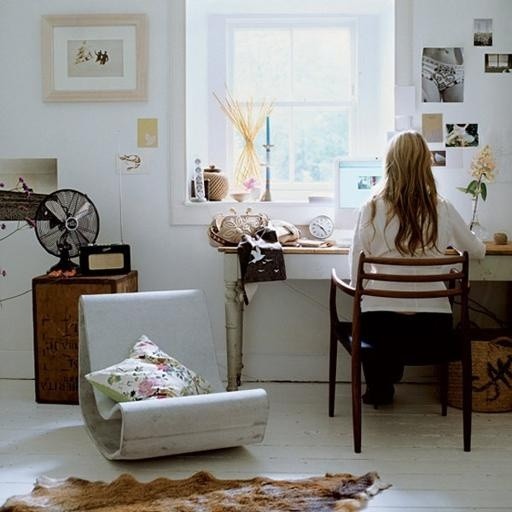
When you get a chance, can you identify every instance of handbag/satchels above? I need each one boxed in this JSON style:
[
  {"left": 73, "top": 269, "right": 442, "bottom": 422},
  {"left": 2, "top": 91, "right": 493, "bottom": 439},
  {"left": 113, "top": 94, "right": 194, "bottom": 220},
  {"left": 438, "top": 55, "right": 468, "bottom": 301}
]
[{"left": 207, "top": 209, "right": 271, "bottom": 247}]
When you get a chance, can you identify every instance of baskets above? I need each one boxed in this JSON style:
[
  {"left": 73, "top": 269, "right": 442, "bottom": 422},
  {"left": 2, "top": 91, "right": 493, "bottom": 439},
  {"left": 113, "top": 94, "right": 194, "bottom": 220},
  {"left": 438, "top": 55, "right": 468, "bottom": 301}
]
[{"left": 447, "top": 334, "right": 512, "bottom": 414}]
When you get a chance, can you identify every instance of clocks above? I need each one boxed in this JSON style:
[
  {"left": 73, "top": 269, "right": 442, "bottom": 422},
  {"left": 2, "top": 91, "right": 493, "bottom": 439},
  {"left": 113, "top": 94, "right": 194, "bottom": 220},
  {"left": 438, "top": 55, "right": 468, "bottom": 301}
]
[{"left": 308, "top": 216, "right": 335, "bottom": 239}]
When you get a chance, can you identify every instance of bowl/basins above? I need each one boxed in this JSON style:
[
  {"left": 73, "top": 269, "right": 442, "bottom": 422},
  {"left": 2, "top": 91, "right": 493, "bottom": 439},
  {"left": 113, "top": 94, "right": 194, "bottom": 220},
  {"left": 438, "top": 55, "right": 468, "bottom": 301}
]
[{"left": 231, "top": 192, "right": 248, "bottom": 203}]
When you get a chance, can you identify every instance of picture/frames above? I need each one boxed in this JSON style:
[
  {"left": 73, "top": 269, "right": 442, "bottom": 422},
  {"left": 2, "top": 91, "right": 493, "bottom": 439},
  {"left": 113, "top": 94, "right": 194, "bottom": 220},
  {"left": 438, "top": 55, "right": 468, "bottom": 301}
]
[{"left": 40, "top": 13, "right": 149, "bottom": 103}]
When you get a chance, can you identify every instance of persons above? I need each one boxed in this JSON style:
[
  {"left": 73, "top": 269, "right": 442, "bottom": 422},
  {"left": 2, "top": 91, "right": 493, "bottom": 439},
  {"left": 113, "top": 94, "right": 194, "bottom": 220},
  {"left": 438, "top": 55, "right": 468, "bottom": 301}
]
[
  {"left": 349, "top": 130, "right": 486, "bottom": 409},
  {"left": 422, "top": 47, "right": 464, "bottom": 102}
]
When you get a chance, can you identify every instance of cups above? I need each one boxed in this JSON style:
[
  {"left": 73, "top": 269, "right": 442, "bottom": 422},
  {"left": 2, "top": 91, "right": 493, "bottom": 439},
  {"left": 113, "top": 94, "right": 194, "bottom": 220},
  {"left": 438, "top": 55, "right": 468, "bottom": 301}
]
[{"left": 493, "top": 232, "right": 507, "bottom": 245}]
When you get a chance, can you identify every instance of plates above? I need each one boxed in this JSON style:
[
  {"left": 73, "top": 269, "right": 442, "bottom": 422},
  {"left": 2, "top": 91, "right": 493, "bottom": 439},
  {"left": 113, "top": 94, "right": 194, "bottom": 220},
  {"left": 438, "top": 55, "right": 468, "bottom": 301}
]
[{"left": 308, "top": 197, "right": 331, "bottom": 203}]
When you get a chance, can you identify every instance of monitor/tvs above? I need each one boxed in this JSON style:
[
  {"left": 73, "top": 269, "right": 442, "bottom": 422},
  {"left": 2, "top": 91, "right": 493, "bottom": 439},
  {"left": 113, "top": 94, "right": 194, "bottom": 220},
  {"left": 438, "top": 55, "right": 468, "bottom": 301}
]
[{"left": 332, "top": 155, "right": 385, "bottom": 230}]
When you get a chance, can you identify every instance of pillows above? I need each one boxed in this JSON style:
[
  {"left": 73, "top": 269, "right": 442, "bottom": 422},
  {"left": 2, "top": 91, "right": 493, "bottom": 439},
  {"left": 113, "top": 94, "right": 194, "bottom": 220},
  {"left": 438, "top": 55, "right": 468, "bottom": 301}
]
[{"left": 83, "top": 333, "right": 212, "bottom": 402}]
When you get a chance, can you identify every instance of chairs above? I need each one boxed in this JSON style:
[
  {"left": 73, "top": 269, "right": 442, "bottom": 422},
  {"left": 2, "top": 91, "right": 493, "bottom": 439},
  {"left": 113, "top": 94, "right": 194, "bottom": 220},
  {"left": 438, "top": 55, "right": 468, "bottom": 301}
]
[
  {"left": 77, "top": 288, "right": 269, "bottom": 462},
  {"left": 326, "top": 249, "right": 474, "bottom": 453}
]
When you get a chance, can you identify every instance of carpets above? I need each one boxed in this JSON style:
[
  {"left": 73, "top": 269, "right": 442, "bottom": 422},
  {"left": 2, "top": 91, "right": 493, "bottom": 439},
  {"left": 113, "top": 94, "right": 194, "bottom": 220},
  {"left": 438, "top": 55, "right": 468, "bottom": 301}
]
[{"left": 1, "top": 470, "right": 394, "bottom": 512}]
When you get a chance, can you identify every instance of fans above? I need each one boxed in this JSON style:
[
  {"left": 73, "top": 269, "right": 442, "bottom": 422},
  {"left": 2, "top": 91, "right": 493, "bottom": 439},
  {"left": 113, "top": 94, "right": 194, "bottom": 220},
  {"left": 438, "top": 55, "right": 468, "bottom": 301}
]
[{"left": 35, "top": 189, "right": 100, "bottom": 274}]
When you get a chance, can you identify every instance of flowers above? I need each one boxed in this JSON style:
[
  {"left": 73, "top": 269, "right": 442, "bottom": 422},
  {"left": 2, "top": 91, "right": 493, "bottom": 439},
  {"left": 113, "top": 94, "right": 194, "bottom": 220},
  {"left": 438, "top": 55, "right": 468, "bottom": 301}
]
[{"left": 455, "top": 143, "right": 498, "bottom": 233}]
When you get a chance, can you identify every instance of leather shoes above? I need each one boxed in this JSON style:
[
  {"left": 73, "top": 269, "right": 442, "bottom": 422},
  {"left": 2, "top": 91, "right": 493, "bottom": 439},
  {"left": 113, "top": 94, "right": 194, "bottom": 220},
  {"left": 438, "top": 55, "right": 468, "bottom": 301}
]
[{"left": 360, "top": 394, "right": 393, "bottom": 409}]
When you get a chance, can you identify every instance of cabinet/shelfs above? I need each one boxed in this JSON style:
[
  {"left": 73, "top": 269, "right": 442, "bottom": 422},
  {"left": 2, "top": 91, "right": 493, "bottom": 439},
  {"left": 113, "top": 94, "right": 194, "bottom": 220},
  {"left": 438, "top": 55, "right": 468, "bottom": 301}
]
[{"left": 31, "top": 271, "right": 138, "bottom": 405}]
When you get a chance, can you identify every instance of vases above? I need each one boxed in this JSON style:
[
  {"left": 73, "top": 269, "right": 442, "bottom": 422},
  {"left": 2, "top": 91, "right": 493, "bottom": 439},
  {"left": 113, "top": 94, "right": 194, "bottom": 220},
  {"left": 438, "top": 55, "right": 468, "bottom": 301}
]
[{"left": 467, "top": 199, "right": 485, "bottom": 241}]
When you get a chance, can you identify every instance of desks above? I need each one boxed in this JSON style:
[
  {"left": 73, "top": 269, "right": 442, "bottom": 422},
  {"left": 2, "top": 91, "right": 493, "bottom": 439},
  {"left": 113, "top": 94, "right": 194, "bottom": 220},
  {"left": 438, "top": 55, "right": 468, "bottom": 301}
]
[{"left": 217, "top": 241, "right": 512, "bottom": 391}]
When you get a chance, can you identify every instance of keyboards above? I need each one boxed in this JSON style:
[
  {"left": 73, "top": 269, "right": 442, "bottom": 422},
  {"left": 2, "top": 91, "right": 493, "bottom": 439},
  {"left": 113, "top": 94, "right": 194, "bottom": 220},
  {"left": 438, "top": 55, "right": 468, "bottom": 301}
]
[{"left": 336, "top": 237, "right": 352, "bottom": 248}]
